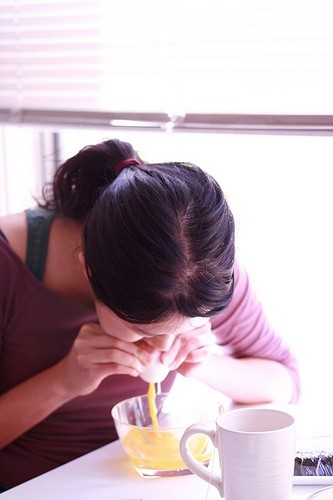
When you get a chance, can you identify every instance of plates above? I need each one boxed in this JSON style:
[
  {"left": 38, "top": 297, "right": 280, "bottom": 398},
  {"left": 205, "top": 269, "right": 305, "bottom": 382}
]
[
  {"left": 293, "top": 476, "right": 333, "bottom": 485},
  {"left": 304, "top": 486, "right": 333, "bottom": 500}
]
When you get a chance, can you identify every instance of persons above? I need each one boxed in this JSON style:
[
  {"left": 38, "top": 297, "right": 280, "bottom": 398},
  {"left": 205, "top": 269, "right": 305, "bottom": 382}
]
[{"left": 0, "top": 139, "right": 300, "bottom": 494}]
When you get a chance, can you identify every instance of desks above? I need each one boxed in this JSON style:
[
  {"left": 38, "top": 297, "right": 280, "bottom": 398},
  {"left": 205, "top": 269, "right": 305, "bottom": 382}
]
[{"left": 0, "top": 403, "right": 333, "bottom": 500}]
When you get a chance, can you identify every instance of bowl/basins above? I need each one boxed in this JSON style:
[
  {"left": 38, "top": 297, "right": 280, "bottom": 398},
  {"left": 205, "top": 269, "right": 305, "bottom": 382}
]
[{"left": 110, "top": 392, "right": 224, "bottom": 479}]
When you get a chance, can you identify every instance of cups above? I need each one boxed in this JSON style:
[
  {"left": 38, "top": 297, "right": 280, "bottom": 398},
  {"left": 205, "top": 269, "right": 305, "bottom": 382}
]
[{"left": 179, "top": 408, "right": 297, "bottom": 500}]
